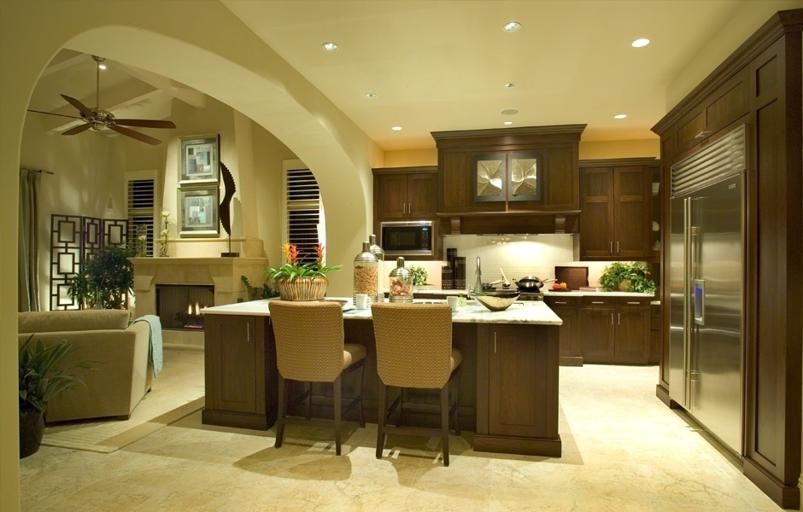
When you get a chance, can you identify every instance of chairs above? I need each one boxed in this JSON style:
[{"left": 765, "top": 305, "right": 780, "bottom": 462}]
[
  {"left": 264, "top": 295, "right": 365, "bottom": 456},
  {"left": 370, "top": 300, "right": 465, "bottom": 468}
]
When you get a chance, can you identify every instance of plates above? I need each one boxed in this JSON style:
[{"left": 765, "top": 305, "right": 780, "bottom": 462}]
[{"left": 548, "top": 289, "right": 571, "bottom": 292}]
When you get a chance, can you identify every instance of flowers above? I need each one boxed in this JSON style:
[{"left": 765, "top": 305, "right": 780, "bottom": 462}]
[{"left": 262, "top": 241, "right": 338, "bottom": 283}]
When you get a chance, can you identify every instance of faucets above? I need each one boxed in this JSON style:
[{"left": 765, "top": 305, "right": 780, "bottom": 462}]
[{"left": 475, "top": 257, "right": 482, "bottom": 291}]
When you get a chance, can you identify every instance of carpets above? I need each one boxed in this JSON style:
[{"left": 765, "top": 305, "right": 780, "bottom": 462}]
[{"left": 39, "top": 346, "right": 205, "bottom": 456}]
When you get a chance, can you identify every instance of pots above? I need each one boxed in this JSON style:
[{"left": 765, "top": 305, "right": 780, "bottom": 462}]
[{"left": 514, "top": 276, "right": 558, "bottom": 290}]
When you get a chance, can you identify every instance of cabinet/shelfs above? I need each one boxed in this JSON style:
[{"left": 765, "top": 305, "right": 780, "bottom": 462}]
[
  {"left": 432, "top": 123, "right": 586, "bottom": 219},
  {"left": 379, "top": 290, "right": 663, "bottom": 369},
  {"left": 577, "top": 160, "right": 662, "bottom": 262},
  {"left": 373, "top": 164, "right": 440, "bottom": 221}
]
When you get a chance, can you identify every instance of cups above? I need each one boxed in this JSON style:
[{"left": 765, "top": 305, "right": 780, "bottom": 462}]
[
  {"left": 355, "top": 292, "right": 371, "bottom": 310},
  {"left": 446, "top": 295, "right": 458, "bottom": 311}
]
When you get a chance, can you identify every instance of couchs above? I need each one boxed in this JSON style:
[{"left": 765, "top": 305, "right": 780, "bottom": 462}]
[{"left": 18, "top": 304, "right": 153, "bottom": 425}]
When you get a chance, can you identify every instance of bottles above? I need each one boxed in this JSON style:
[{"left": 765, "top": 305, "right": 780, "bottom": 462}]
[
  {"left": 352, "top": 241, "right": 378, "bottom": 306},
  {"left": 440, "top": 247, "right": 466, "bottom": 290},
  {"left": 368, "top": 233, "right": 386, "bottom": 306},
  {"left": 387, "top": 256, "right": 414, "bottom": 303}
]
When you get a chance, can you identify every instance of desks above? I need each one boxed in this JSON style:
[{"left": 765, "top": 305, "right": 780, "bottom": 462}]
[{"left": 193, "top": 292, "right": 565, "bottom": 460}]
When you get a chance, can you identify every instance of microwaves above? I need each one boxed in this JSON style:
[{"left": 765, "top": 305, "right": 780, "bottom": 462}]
[{"left": 379, "top": 220, "right": 435, "bottom": 257}]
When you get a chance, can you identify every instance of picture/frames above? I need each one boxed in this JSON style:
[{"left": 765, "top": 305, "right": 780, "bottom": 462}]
[
  {"left": 175, "top": 186, "right": 219, "bottom": 239},
  {"left": 175, "top": 131, "right": 221, "bottom": 185}
]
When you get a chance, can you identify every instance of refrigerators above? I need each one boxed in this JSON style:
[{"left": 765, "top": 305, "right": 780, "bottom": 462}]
[{"left": 667, "top": 125, "right": 745, "bottom": 468}]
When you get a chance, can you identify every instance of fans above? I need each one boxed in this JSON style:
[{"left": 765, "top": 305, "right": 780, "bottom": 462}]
[{"left": 22, "top": 54, "right": 176, "bottom": 147}]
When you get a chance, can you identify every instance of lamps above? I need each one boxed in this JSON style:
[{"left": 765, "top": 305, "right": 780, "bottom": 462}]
[
  {"left": 392, "top": 126, "right": 403, "bottom": 131},
  {"left": 504, "top": 121, "right": 513, "bottom": 125}
]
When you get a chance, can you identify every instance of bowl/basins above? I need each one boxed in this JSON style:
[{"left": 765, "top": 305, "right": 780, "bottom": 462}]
[{"left": 473, "top": 293, "right": 520, "bottom": 312}]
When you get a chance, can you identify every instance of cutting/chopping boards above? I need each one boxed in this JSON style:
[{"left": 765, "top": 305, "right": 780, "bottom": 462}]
[{"left": 555, "top": 266, "right": 587, "bottom": 290}]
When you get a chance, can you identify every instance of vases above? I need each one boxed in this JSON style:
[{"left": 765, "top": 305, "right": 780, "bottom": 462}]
[{"left": 274, "top": 276, "right": 328, "bottom": 300}]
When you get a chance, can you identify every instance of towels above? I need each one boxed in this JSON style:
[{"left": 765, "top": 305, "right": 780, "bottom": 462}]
[{"left": 130, "top": 313, "right": 163, "bottom": 379}]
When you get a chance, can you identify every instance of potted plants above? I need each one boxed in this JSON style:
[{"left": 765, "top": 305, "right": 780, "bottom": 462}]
[
  {"left": 591, "top": 258, "right": 658, "bottom": 297},
  {"left": 17, "top": 326, "right": 112, "bottom": 459}
]
